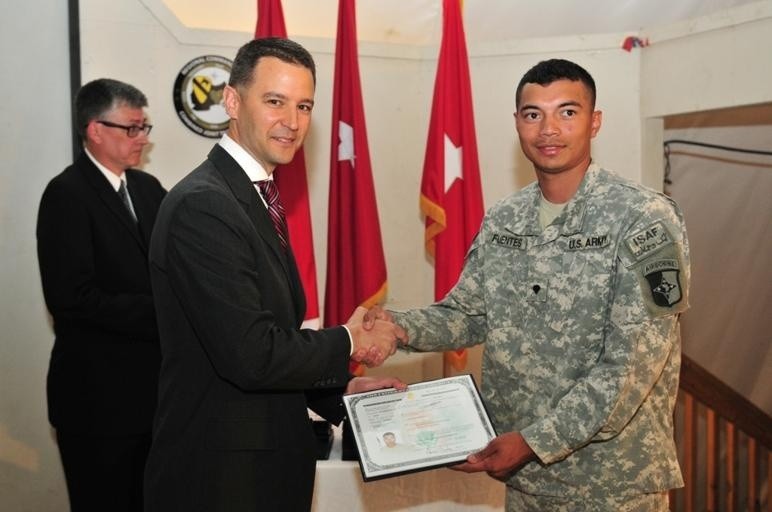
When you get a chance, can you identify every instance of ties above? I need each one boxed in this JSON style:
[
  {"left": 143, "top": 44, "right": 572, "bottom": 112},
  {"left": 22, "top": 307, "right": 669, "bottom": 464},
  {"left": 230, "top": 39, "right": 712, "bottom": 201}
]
[
  {"left": 254, "top": 179, "right": 289, "bottom": 255},
  {"left": 117, "top": 179, "right": 136, "bottom": 223}
]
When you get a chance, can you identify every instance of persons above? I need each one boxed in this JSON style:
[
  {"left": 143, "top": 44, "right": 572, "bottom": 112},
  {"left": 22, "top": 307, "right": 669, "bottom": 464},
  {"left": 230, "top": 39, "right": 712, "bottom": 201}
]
[
  {"left": 147, "top": 38, "right": 407, "bottom": 512},
  {"left": 351, "top": 58, "right": 691, "bottom": 512},
  {"left": 36, "top": 79, "right": 168, "bottom": 512}
]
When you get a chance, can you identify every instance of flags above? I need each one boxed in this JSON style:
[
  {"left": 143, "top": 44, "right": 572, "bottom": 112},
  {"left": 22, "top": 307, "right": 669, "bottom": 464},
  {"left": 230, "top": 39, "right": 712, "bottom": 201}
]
[
  {"left": 255, "top": 0, "right": 320, "bottom": 331},
  {"left": 324, "top": 0, "right": 387, "bottom": 377},
  {"left": 419, "top": 0, "right": 484, "bottom": 371}
]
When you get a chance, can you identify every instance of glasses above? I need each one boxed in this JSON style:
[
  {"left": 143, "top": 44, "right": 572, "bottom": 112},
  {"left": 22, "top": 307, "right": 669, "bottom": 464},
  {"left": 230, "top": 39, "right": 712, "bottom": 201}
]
[{"left": 95, "top": 120, "right": 153, "bottom": 138}]
